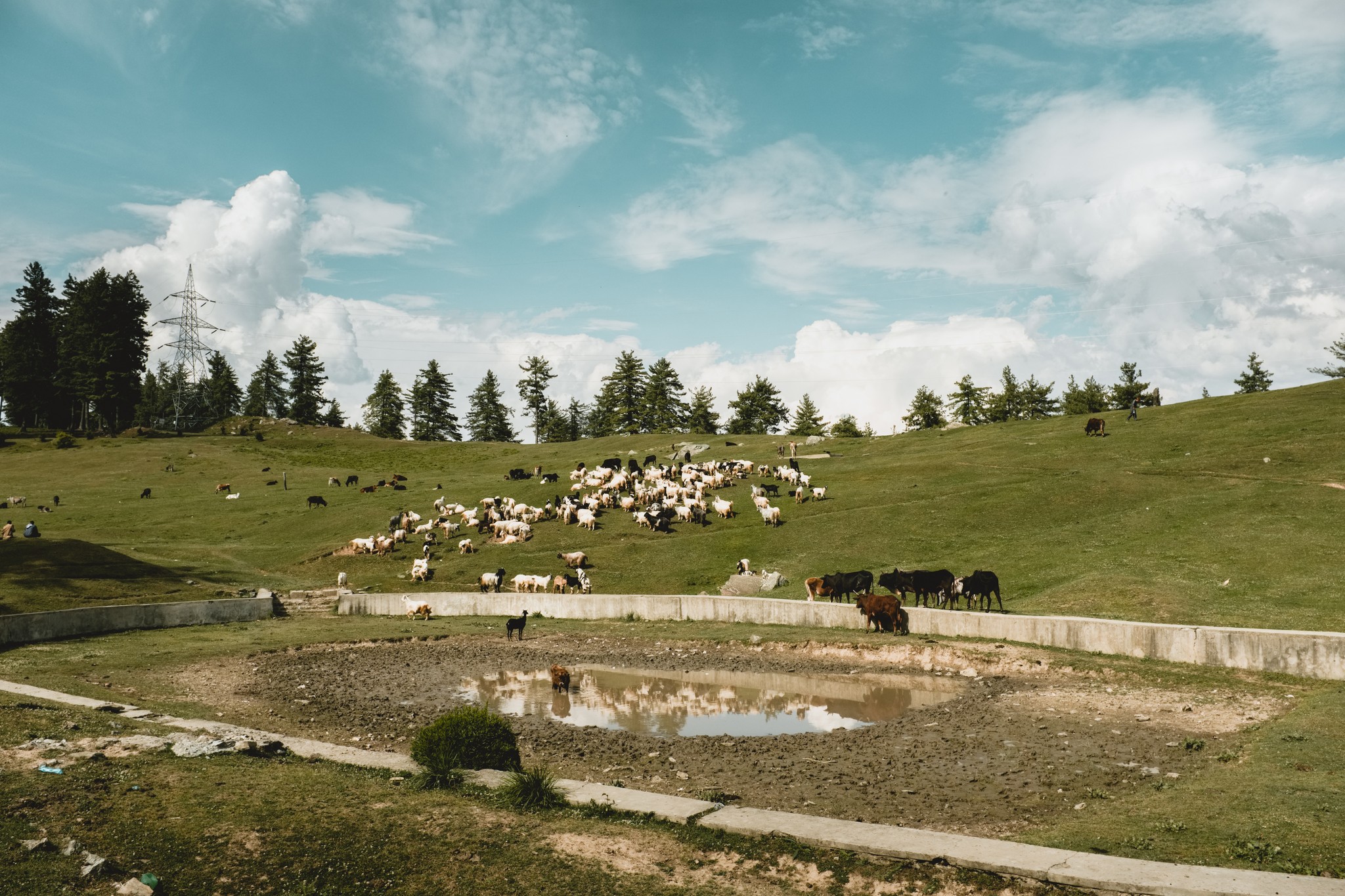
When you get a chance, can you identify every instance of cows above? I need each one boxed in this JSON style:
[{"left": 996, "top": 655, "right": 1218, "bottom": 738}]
[
  {"left": 140, "top": 488, "right": 151, "bottom": 499},
  {"left": 803, "top": 567, "right": 1007, "bottom": 638},
  {"left": 7, "top": 495, "right": 27, "bottom": 508},
  {"left": 506, "top": 608, "right": 529, "bottom": 642},
  {"left": 550, "top": 664, "right": 571, "bottom": 693},
  {"left": 53, "top": 495, "right": 60, "bottom": 507},
  {"left": 262, "top": 467, "right": 271, "bottom": 472},
  {"left": 266, "top": 480, "right": 278, "bottom": 486},
  {"left": 359, "top": 473, "right": 408, "bottom": 493},
  {"left": 328, "top": 475, "right": 359, "bottom": 488},
  {"left": 0, "top": 502, "right": 8, "bottom": 509},
  {"left": 432, "top": 484, "right": 443, "bottom": 490},
  {"left": 1081, "top": 417, "right": 1105, "bottom": 438},
  {"left": 166, "top": 463, "right": 174, "bottom": 472},
  {"left": 37, "top": 505, "right": 51, "bottom": 513},
  {"left": 214, "top": 483, "right": 241, "bottom": 500},
  {"left": 306, "top": 496, "right": 329, "bottom": 510},
  {"left": 503, "top": 465, "right": 543, "bottom": 481}
]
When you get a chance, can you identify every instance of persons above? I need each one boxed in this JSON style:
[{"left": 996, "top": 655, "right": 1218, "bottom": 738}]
[
  {"left": 623, "top": 431, "right": 631, "bottom": 437},
  {"left": 1126, "top": 398, "right": 1140, "bottom": 422},
  {"left": 24, "top": 520, "right": 41, "bottom": 538},
  {"left": 1, "top": 520, "right": 16, "bottom": 539}
]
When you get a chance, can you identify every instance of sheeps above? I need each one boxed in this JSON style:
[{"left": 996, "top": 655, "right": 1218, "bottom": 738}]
[
  {"left": 761, "top": 569, "right": 772, "bottom": 579},
  {"left": 569, "top": 438, "right": 828, "bottom": 534},
  {"left": 736, "top": 558, "right": 756, "bottom": 577},
  {"left": 348, "top": 489, "right": 600, "bottom": 594},
  {"left": 400, "top": 595, "right": 433, "bottom": 621},
  {"left": 337, "top": 571, "right": 348, "bottom": 591},
  {"left": 540, "top": 473, "right": 560, "bottom": 484}
]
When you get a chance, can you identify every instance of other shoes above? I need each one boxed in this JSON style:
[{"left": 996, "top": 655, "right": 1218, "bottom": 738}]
[{"left": 1126, "top": 418, "right": 1130, "bottom": 422}]
